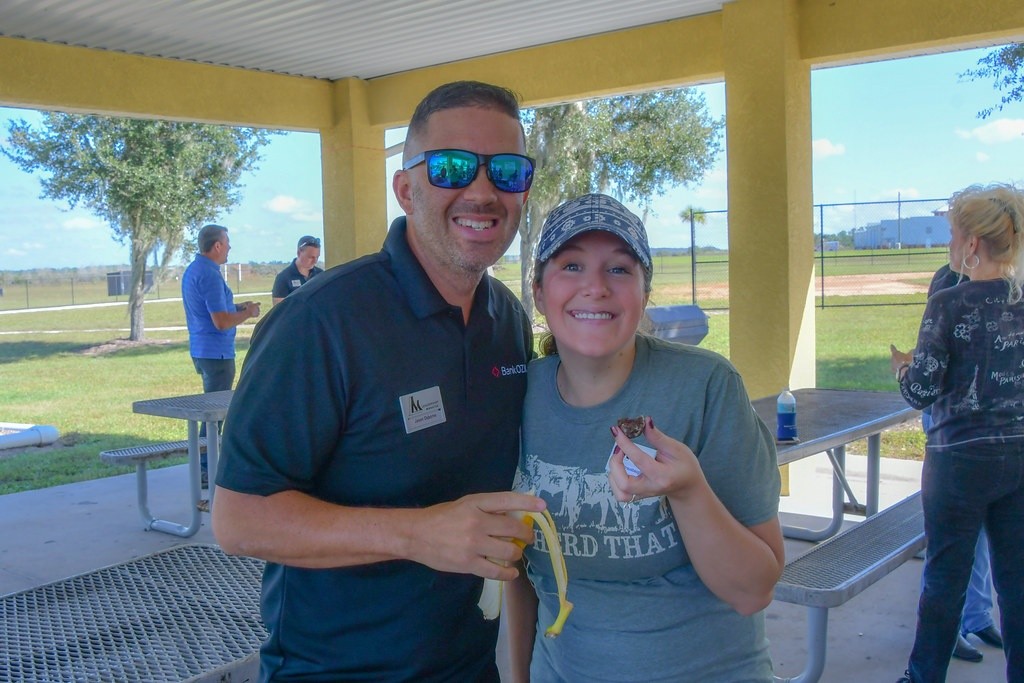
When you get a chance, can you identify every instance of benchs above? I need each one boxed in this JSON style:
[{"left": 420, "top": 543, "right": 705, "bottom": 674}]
[
  {"left": 772, "top": 489, "right": 927, "bottom": 682},
  {"left": 99, "top": 438, "right": 223, "bottom": 532}
]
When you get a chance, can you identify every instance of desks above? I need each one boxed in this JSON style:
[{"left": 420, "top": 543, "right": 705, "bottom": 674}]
[
  {"left": 750, "top": 389, "right": 921, "bottom": 540},
  {"left": 132, "top": 389, "right": 234, "bottom": 538},
  {"left": 0, "top": 544, "right": 268, "bottom": 683}
]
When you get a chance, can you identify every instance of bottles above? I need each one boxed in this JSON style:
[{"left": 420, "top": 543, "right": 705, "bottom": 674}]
[{"left": 776, "top": 387, "right": 798, "bottom": 441}]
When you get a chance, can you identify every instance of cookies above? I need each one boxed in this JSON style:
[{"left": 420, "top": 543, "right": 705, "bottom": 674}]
[{"left": 618, "top": 415, "right": 645, "bottom": 438}]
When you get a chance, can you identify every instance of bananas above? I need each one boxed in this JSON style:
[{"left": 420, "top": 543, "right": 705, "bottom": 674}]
[{"left": 478, "top": 488, "right": 573, "bottom": 638}]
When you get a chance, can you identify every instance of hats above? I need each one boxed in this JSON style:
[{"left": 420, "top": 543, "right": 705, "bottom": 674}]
[{"left": 538, "top": 194, "right": 651, "bottom": 268}]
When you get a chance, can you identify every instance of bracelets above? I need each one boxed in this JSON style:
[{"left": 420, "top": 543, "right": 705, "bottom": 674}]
[{"left": 895, "top": 362, "right": 911, "bottom": 383}]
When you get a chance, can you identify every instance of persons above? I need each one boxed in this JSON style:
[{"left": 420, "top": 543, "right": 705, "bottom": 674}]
[
  {"left": 506, "top": 192, "right": 785, "bottom": 683},
  {"left": 440, "top": 165, "right": 475, "bottom": 184},
  {"left": 211, "top": 80, "right": 547, "bottom": 683},
  {"left": 272, "top": 236, "right": 325, "bottom": 304},
  {"left": 493, "top": 167, "right": 531, "bottom": 190},
  {"left": 921, "top": 262, "right": 1003, "bottom": 663},
  {"left": 181, "top": 225, "right": 261, "bottom": 488},
  {"left": 890, "top": 184, "right": 1024, "bottom": 683}
]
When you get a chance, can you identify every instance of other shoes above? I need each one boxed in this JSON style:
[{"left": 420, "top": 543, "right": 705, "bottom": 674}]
[
  {"left": 952, "top": 634, "right": 983, "bottom": 661},
  {"left": 200, "top": 481, "right": 208, "bottom": 489},
  {"left": 963, "top": 624, "right": 1002, "bottom": 649},
  {"left": 895, "top": 669, "right": 911, "bottom": 683}
]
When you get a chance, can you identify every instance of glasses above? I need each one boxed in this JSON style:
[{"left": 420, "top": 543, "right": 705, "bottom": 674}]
[
  {"left": 299, "top": 238, "right": 320, "bottom": 248},
  {"left": 403, "top": 149, "right": 537, "bottom": 193}
]
